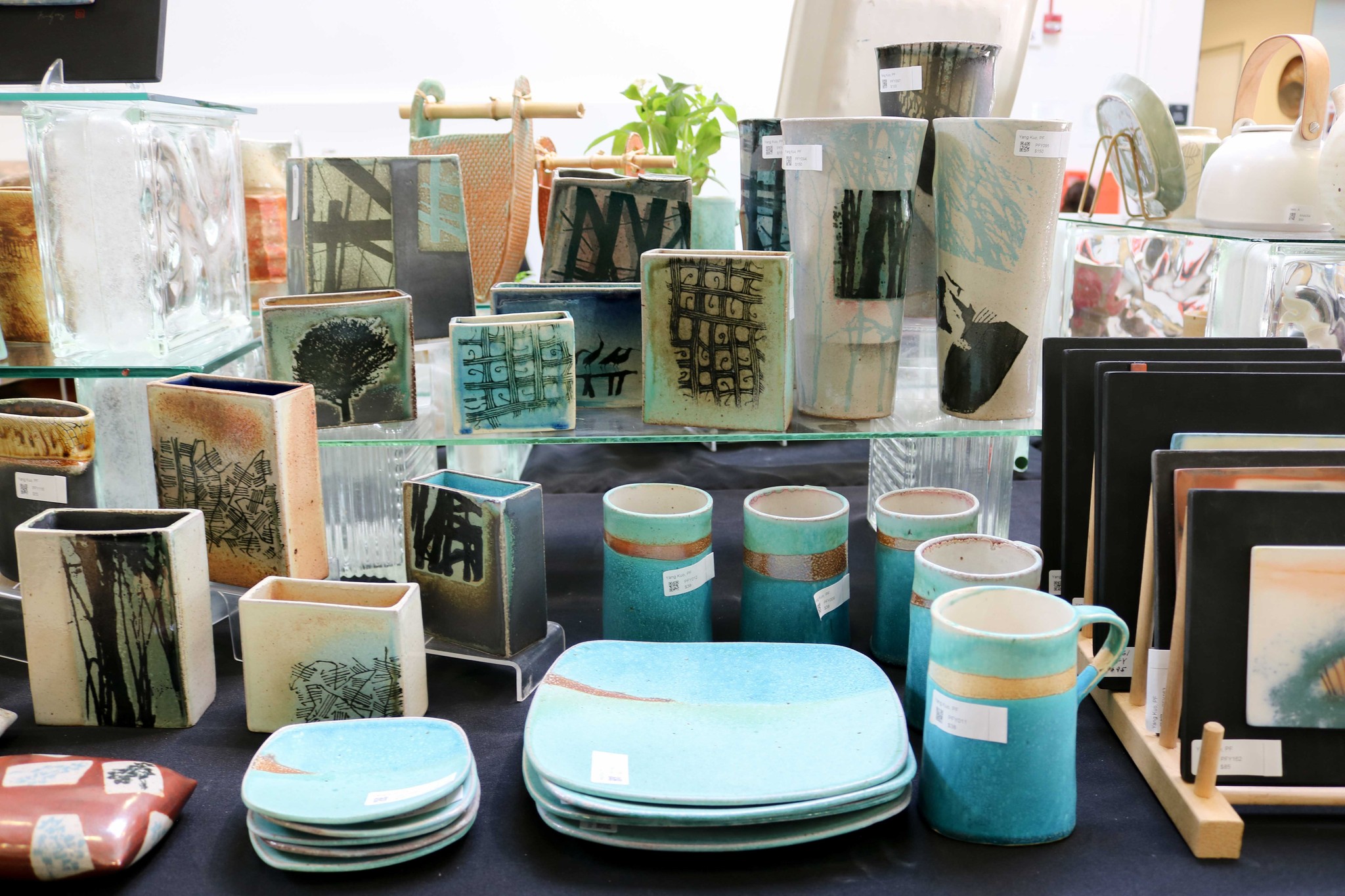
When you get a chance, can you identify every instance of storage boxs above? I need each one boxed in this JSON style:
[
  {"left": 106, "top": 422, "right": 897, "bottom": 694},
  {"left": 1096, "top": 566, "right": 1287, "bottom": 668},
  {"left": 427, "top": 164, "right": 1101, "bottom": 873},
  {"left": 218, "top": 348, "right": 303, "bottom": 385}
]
[
  {"left": 1, "top": 84, "right": 260, "bottom": 356},
  {"left": 1061, "top": 210, "right": 1345, "bottom": 350}
]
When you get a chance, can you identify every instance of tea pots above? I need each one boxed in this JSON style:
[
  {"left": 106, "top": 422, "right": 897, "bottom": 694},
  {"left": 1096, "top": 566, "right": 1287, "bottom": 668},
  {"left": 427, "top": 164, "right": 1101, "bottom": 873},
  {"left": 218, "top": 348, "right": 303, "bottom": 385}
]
[{"left": 1196, "top": 34, "right": 1345, "bottom": 236}]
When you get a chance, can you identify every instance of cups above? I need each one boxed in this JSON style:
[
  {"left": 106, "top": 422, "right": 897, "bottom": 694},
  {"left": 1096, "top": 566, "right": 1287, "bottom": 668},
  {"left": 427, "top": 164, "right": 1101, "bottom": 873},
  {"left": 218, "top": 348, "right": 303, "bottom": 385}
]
[
  {"left": 1184, "top": 310, "right": 1208, "bottom": 337},
  {"left": 907, "top": 534, "right": 1044, "bottom": 711},
  {"left": 933, "top": 117, "right": 1074, "bottom": 423},
  {"left": 870, "top": 487, "right": 980, "bottom": 667},
  {"left": 602, "top": 483, "right": 715, "bottom": 642},
  {"left": 742, "top": 484, "right": 850, "bottom": 649},
  {"left": 739, "top": 116, "right": 929, "bottom": 419},
  {"left": 917, "top": 586, "right": 1129, "bottom": 845}
]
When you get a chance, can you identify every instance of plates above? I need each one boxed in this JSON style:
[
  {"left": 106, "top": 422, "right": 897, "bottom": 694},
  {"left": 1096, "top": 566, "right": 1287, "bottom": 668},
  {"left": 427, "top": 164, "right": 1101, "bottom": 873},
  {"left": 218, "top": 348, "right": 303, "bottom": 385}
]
[
  {"left": 522, "top": 639, "right": 917, "bottom": 851},
  {"left": 240, "top": 717, "right": 481, "bottom": 872},
  {"left": 1096, "top": 73, "right": 1186, "bottom": 211}
]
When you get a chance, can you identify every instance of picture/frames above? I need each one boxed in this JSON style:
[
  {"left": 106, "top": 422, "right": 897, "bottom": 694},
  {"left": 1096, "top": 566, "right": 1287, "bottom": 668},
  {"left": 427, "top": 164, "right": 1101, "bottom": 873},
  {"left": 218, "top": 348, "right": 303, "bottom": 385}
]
[{"left": 1182, "top": 488, "right": 1344, "bottom": 786}]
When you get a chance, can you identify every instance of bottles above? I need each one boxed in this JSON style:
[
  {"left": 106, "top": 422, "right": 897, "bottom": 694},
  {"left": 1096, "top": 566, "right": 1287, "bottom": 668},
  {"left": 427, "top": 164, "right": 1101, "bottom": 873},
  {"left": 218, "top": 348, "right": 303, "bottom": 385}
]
[{"left": 1174, "top": 126, "right": 1221, "bottom": 217}]
